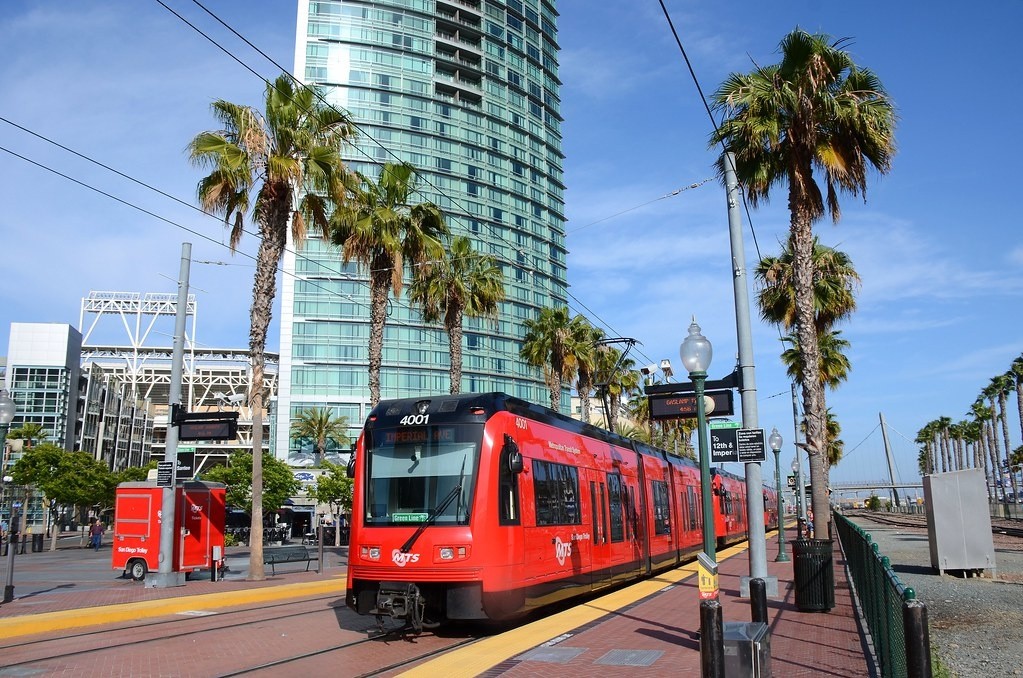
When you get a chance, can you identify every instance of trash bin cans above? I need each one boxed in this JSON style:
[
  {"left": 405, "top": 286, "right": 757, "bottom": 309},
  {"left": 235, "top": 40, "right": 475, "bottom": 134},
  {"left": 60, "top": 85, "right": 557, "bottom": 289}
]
[
  {"left": 8, "top": 534, "right": 19, "bottom": 554},
  {"left": 814, "top": 522, "right": 832, "bottom": 539},
  {"left": 789, "top": 538, "right": 836, "bottom": 614},
  {"left": 32, "top": 534, "right": 44, "bottom": 552}
]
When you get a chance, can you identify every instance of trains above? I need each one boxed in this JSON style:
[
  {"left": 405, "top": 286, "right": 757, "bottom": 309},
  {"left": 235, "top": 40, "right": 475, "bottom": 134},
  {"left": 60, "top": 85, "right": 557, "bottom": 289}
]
[{"left": 342, "top": 391, "right": 779, "bottom": 636}]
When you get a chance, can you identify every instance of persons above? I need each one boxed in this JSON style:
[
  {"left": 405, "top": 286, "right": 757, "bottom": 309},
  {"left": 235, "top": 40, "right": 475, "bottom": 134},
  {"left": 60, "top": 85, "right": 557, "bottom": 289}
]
[
  {"left": 88, "top": 519, "right": 105, "bottom": 552},
  {"left": 0, "top": 521, "right": 8, "bottom": 539}
]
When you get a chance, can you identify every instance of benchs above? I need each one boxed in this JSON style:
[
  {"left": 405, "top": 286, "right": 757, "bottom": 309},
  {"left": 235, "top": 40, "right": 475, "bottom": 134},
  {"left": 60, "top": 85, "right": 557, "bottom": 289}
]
[{"left": 263, "top": 545, "right": 318, "bottom": 576}]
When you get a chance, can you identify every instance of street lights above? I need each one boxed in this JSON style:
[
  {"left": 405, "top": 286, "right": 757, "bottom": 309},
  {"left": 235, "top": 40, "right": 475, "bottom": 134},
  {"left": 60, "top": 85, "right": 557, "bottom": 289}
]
[
  {"left": 680, "top": 313, "right": 725, "bottom": 678},
  {"left": 769, "top": 428, "right": 791, "bottom": 563},
  {"left": 0, "top": 389, "right": 16, "bottom": 479},
  {"left": 791, "top": 456, "right": 803, "bottom": 540}
]
